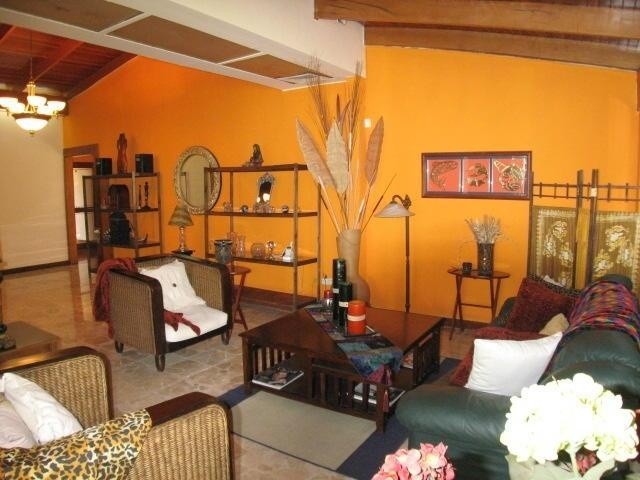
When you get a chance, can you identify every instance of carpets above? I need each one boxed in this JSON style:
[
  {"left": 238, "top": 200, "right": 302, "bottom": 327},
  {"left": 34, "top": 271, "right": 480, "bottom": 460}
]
[{"left": 219, "top": 355, "right": 463, "bottom": 480}]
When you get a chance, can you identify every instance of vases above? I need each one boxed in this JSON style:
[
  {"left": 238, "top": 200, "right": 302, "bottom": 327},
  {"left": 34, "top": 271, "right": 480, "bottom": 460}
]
[
  {"left": 477, "top": 244, "right": 492, "bottom": 276},
  {"left": 336, "top": 229, "right": 370, "bottom": 307}
]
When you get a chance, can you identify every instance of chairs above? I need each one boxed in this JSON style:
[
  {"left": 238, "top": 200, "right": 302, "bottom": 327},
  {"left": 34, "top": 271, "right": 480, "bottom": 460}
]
[
  {"left": 107, "top": 253, "right": 232, "bottom": 372},
  {"left": 0, "top": 347, "right": 235, "bottom": 479}
]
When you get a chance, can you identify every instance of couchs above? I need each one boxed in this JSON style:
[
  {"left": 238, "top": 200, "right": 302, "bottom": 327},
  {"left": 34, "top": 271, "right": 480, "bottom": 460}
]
[{"left": 383, "top": 274, "right": 640, "bottom": 480}]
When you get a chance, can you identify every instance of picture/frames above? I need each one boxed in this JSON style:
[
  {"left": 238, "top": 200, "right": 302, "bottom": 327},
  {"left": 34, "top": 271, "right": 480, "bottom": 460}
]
[{"left": 421, "top": 150, "right": 532, "bottom": 201}]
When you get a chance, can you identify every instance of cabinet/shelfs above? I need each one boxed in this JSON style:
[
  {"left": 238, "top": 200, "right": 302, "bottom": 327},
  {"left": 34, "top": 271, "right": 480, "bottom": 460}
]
[
  {"left": 203, "top": 164, "right": 321, "bottom": 312},
  {"left": 82, "top": 173, "right": 163, "bottom": 288}
]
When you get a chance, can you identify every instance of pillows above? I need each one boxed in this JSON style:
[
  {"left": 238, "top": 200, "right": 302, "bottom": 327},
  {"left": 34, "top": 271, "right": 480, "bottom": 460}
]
[
  {"left": 138, "top": 260, "right": 205, "bottom": 310},
  {"left": 448, "top": 279, "right": 575, "bottom": 397},
  {"left": 1, "top": 372, "right": 84, "bottom": 445},
  {"left": 1, "top": 401, "right": 34, "bottom": 452}
]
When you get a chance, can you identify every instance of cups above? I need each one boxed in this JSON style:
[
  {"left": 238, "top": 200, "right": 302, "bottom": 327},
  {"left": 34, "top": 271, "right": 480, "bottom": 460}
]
[
  {"left": 462, "top": 262, "right": 472, "bottom": 275},
  {"left": 346, "top": 300, "right": 367, "bottom": 334},
  {"left": 236, "top": 235, "right": 247, "bottom": 256}
]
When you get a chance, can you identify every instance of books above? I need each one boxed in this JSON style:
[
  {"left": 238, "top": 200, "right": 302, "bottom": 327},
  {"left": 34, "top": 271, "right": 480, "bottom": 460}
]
[
  {"left": 252, "top": 367, "right": 304, "bottom": 391},
  {"left": 349, "top": 383, "right": 405, "bottom": 406}
]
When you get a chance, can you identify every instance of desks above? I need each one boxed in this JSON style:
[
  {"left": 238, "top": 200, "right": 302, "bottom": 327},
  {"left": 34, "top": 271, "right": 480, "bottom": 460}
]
[
  {"left": 447, "top": 268, "right": 510, "bottom": 339},
  {"left": 0, "top": 320, "right": 61, "bottom": 361},
  {"left": 224, "top": 265, "right": 251, "bottom": 344}
]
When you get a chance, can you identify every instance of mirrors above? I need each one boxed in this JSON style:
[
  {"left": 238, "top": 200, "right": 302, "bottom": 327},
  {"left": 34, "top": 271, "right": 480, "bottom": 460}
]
[
  {"left": 254, "top": 174, "right": 275, "bottom": 214},
  {"left": 173, "top": 145, "right": 221, "bottom": 215}
]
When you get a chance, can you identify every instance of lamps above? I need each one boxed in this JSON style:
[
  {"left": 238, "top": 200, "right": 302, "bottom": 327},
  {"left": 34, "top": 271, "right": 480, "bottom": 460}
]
[
  {"left": 0, "top": 30, "right": 67, "bottom": 136},
  {"left": 168, "top": 205, "right": 195, "bottom": 255},
  {"left": 375, "top": 194, "right": 415, "bottom": 312}
]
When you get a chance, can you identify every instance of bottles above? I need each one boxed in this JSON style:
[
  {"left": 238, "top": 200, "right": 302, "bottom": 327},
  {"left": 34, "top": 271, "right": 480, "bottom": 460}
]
[
  {"left": 322, "top": 290, "right": 333, "bottom": 321},
  {"left": 333, "top": 258, "right": 347, "bottom": 323},
  {"left": 338, "top": 283, "right": 353, "bottom": 331}
]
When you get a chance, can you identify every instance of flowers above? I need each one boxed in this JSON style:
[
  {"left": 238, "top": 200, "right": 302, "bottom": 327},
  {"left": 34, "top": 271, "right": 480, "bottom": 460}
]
[
  {"left": 370, "top": 441, "right": 456, "bottom": 480},
  {"left": 499, "top": 372, "right": 640, "bottom": 480}
]
